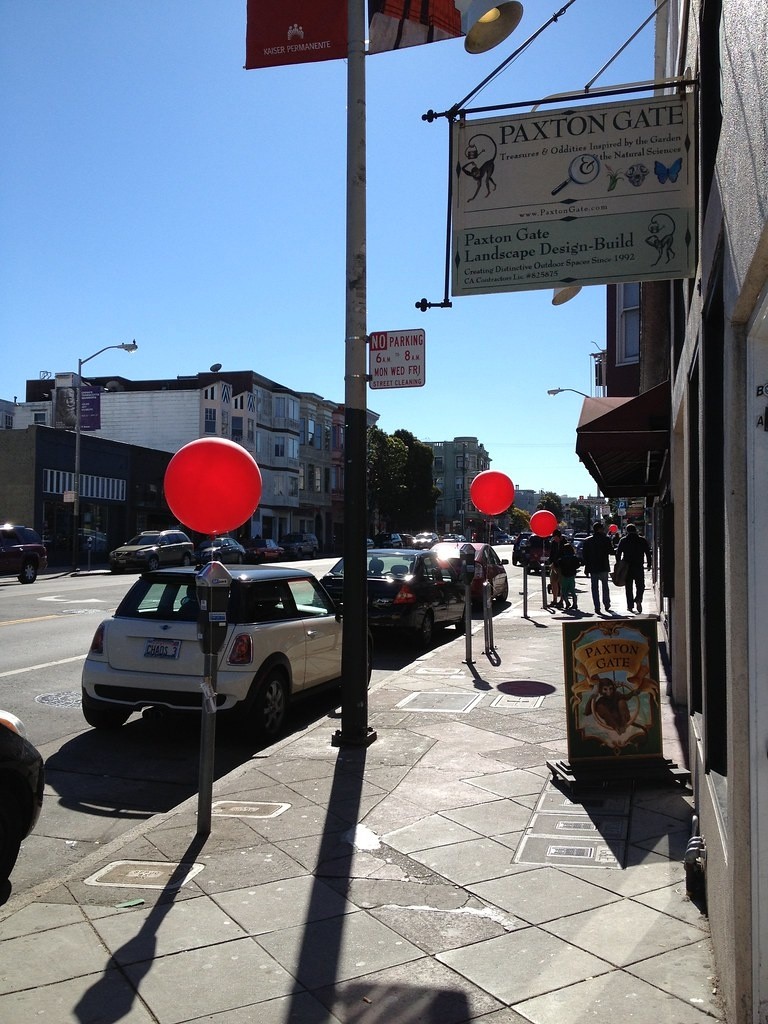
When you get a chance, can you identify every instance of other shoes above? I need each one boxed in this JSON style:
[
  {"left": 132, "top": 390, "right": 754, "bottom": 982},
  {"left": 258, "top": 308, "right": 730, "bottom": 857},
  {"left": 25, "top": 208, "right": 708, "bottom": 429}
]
[
  {"left": 547, "top": 600, "right": 564, "bottom": 608},
  {"left": 565, "top": 602, "right": 570, "bottom": 609},
  {"left": 605, "top": 602, "right": 610, "bottom": 611},
  {"left": 595, "top": 608, "right": 602, "bottom": 615},
  {"left": 569, "top": 604, "right": 577, "bottom": 609},
  {"left": 636, "top": 600, "right": 642, "bottom": 614},
  {"left": 628, "top": 606, "right": 633, "bottom": 611}
]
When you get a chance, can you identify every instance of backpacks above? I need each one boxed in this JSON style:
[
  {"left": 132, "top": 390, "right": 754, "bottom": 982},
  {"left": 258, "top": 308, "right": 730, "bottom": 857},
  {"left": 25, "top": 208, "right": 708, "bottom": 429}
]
[{"left": 582, "top": 539, "right": 597, "bottom": 574}]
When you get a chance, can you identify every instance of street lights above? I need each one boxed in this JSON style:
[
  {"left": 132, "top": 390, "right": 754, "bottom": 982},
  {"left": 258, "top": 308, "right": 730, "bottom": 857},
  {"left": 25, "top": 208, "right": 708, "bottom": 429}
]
[{"left": 67, "top": 344, "right": 139, "bottom": 575}]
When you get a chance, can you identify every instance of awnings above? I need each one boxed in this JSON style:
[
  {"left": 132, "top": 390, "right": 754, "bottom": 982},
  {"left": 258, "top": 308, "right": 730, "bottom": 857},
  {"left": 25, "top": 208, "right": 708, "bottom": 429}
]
[{"left": 576, "top": 380, "right": 671, "bottom": 498}]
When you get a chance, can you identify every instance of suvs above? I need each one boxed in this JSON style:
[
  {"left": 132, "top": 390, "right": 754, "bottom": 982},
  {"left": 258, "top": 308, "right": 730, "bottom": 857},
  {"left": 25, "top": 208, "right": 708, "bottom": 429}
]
[
  {"left": 0, "top": 524, "right": 48, "bottom": 584},
  {"left": 108, "top": 529, "right": 196, "bottom": 575},
  {"left": 278, "top": 532, "right": 320, "bottom": 560}
]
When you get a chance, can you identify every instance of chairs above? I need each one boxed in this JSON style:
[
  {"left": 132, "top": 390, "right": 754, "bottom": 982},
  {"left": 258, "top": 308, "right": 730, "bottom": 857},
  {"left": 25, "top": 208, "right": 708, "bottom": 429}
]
[
  {"left": 367, "top": 559, "right": 384, "bottom": 579},
  {"left": 390, "top": 564, "right": 409, "bottom": 578}
]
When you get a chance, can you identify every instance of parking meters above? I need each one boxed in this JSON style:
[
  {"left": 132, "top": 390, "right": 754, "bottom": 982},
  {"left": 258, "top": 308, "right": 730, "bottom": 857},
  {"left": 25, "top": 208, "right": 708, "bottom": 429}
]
[
  {"left": 461, "top": 544, "right": 476, "bottom": 664},
  {"left": 520, "top": 539, "right": 531, "bottom": 619},
  {"left": 192, "top": 559, "right": 232, "bottom": 832}
]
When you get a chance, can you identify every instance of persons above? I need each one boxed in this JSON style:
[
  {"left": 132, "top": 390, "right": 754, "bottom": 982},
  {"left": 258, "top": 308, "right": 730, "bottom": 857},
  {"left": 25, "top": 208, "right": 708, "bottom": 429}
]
[
  {"left": 619, "top": 523, "right": 652, "bottom": 614},
  {"left": 547, "top": 528, "right": 577, "bottom": 610},
  {"left": 606, "top": 531, "right": 628, "bottom": 558},
  {"left": 583, "top": 521, "right": 616, "bottom": 613},
  {"left": 56, "top": 387, "right": 76, "bottom": 421}
]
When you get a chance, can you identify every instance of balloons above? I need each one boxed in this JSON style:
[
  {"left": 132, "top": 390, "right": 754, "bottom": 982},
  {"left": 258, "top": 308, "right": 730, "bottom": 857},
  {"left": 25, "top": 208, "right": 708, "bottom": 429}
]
[
  {"left": 469, "top": 470, "right": 515, "bottom": 516},
  {"left": 530, "top": 510, "right": 558, "bottom": 538},
  {"left": 609, "top": 524, "right": 618, "bottom": 532},
  {"left": 165, "top": 437, "right": 263, "bottom": 538}
]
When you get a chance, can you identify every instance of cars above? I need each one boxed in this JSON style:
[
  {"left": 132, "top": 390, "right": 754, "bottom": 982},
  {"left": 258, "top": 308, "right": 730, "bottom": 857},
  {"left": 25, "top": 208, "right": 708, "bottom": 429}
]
[
  {"left": 399, "top": 534, "right": 415, "bottom": 548},
  {"left": 442, "top": 533, "right": 459, "bottom": 542},
  {"left": 372, "top": 533, "right": 403, "bottom": 550},
  {"left": 413, "top": 532, "right": 440, "bottom": 550},
  {"left": 309, "top": 547, "right": 467, "bottom": 649},
  {"left": 244, "top": 538, "right": 285, "bottom": 559},
  {"left": 79, "top": 561, "right": 373, "bottom": 740},
  {"left": 511, "top": 530, "right": 592, "bottom": 570},
  {"left": 427, "top": 542, "right": 509, "bottom": 608},
  {"left": 194, "top": 536, "right": 246, "bottom": 564}
]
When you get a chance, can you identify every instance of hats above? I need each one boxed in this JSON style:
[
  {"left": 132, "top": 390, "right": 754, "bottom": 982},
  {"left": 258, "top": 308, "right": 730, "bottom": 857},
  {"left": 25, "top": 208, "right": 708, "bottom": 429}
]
[{"left": 593, "top": 522, "right": 603, "bottom": 531}]
